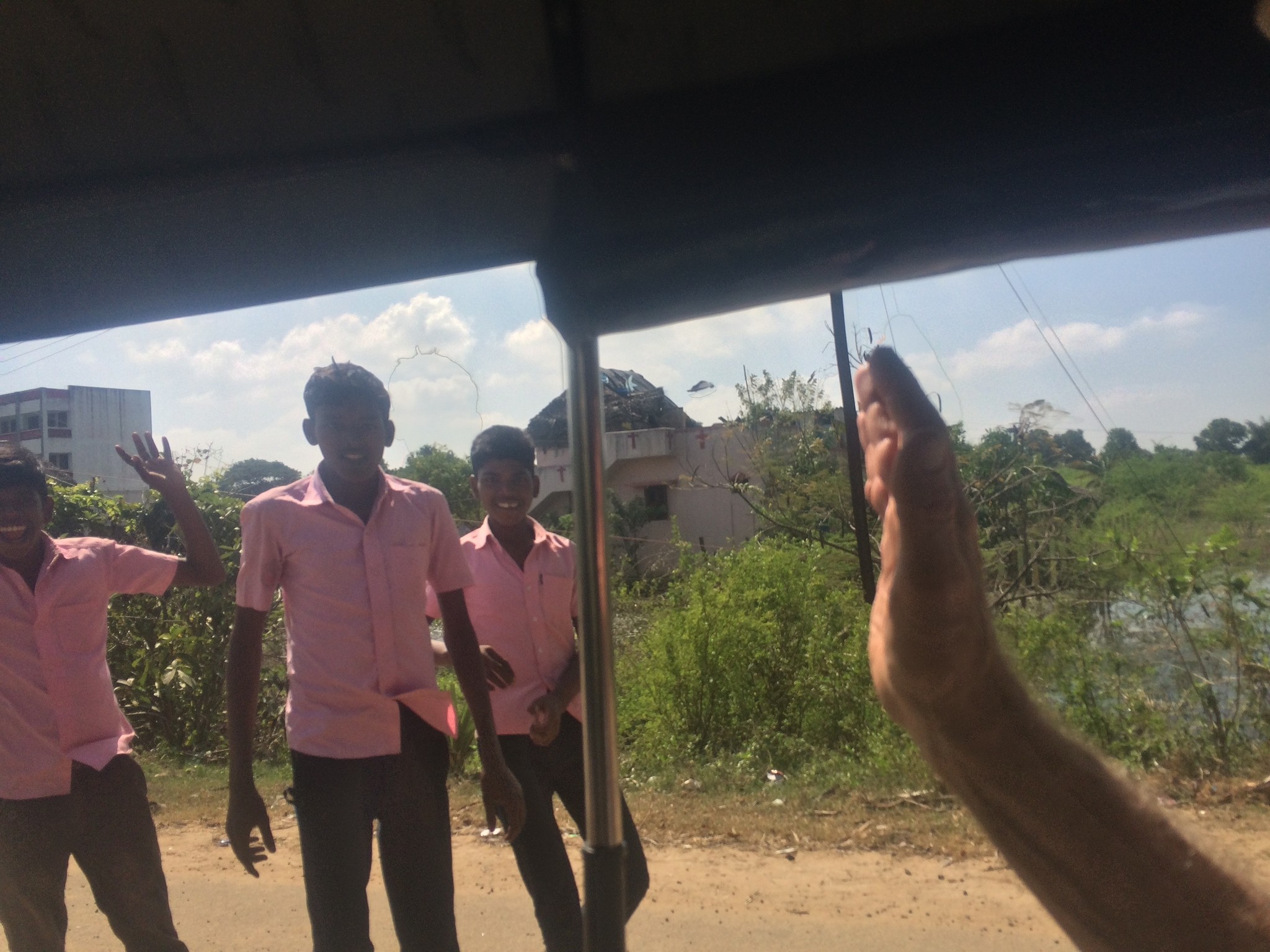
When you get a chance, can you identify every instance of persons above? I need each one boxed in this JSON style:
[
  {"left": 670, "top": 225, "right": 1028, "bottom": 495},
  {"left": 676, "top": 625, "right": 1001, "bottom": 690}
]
[
  {"left": 424, "top": 424, "right": 651, "bottom": 952},
  {"left": 0, "top": 432, "right": 224, "bottom": 952},
  {"left": 223, "top": 355, "right": 528, "bottom": 952},
  {"left": 843, "top": 345, "right": 1270, "bottom": 952}
]
